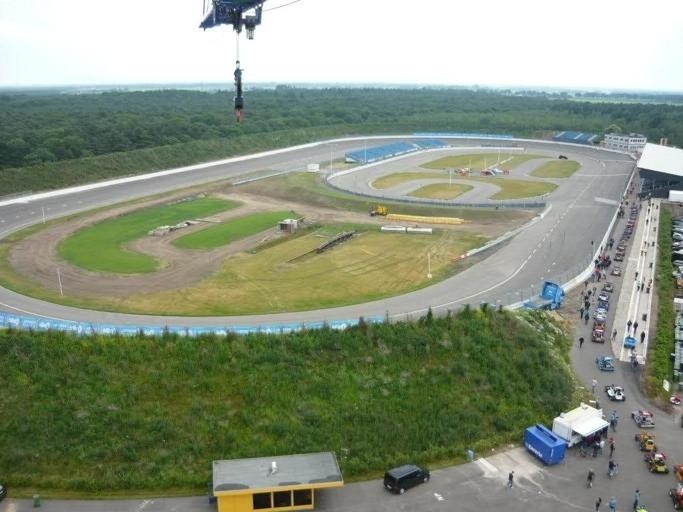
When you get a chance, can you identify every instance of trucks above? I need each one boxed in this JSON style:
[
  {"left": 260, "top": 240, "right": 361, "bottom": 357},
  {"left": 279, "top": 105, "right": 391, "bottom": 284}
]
[{"left": 523, "top": 281, "right": 564, "bottom": 310}]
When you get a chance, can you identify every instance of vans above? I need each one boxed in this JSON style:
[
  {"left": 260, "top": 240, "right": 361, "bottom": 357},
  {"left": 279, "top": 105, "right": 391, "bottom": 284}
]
[{"left": 384, "top": 465, "right": 429, "bottom": 494}]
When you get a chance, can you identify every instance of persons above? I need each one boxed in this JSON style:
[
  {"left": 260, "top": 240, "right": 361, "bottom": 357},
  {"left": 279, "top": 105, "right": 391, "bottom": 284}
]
[
  {"left": 578, "top": 436, "right": 587, "bottom": 458},
  {"left": 605, "top": 496, "right": 617, "bottom": 512},
  {"left": 577, "top": 181, "right": 661, "bottom": 370},
  {"left": 633, "top": 488, "right": 641, "bottom": 509},
  {"left": 634, "top": 504, "right": 648, "bottom": 512},
  {"left": 465, "top": 447, "right": 474, "bottom": 463},
  {"left": 593, "top": 496, "right": 601, "bottom": 512},
  {"left": 590, "top": 409, "right": 620, "bottom": 478},
  {"left": 590, "top": 376, "right": 598, "bottom": 395},
  {"left": 506, "top": 470, "right": 514, "bottom": 490},
  {"left": 586, "top": 467, "right": 595, "bottom": 488}
]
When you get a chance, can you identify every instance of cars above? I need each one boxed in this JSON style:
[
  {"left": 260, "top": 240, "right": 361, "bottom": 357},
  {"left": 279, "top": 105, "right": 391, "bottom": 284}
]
[
  {"left": 671, "top": 204, "right": 683, "bottom": 288},
  {"left": 0, "top": 485, "right": 6, "bottom": 500}
]
